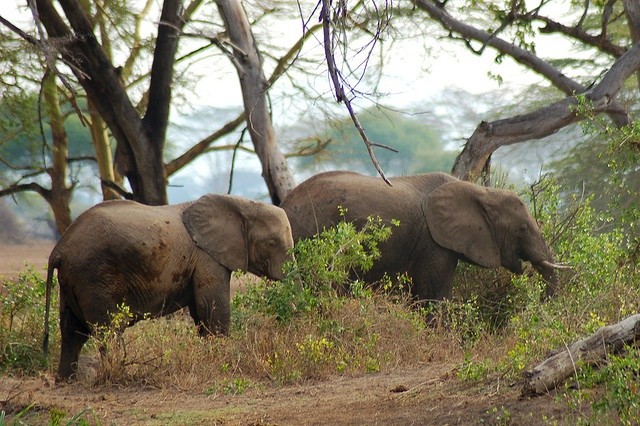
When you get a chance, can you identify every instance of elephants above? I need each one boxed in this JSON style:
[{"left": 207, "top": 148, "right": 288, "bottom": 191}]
[
  {"left": 278, "top": 168, "right": 579, "bottom": 330},
  {"left": 41, "top": 192, "right": 307, "bottom": 388}
]
